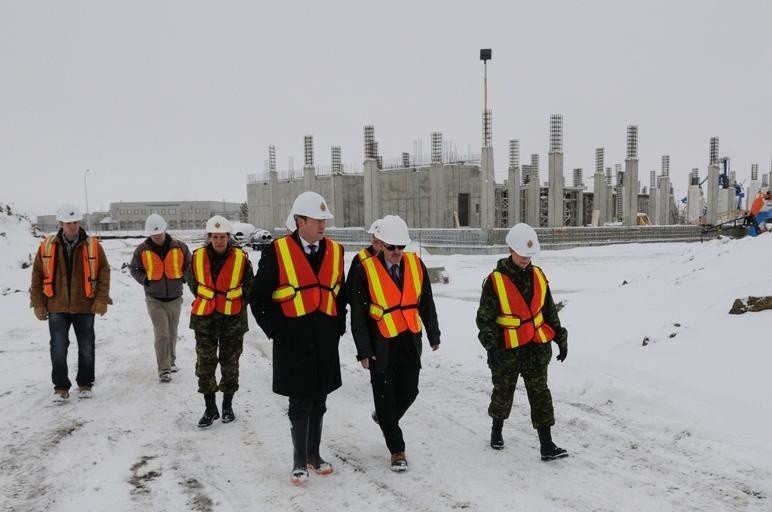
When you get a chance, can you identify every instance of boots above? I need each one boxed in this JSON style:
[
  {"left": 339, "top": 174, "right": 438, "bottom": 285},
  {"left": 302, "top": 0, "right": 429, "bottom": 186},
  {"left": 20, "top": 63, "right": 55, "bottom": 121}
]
[
  {"left": 306, "top": 416, "right": 334, "bottom": 475},
  {"left": 490, "top": 418, "right": 505, "bottom": 450},
  {"left": 198, "top": 392, "right": 220, "bottom": 427},
  {"left": 537, "top": 427, "right": 569, "bottom": 461},
  {"left": 221, "top": 392, "right": 236, "bottom": 424},
  {"left": 289, "top": 416, "right": 310, "bottom": 485}
]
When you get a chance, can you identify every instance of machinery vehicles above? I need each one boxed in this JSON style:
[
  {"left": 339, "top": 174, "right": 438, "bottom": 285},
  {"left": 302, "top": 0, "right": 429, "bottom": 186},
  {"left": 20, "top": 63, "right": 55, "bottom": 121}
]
[{"left": 232, "top": 227, "right": 279, "bottom": 252}]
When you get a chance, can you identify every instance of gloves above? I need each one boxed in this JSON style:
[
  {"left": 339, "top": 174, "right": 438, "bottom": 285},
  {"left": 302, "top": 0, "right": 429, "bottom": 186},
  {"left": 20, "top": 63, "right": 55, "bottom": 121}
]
[
  {"left": 487, "top": 349, "right": 504, "bottom": 372},
  {"left": 556, "top": 339, "right": 568, "bottom": 362},
  {"left": 91, "top": 300, "right": 107, "bottom": 317},
  {"left": 34, "top": 304, "right": 49, "bottom": 321}
]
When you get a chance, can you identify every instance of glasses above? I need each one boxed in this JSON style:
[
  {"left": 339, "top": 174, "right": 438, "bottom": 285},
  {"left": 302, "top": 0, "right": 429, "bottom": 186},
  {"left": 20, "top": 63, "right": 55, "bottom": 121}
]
[{"left": 382, "top": 241, "right": 407, "bottom": 251}]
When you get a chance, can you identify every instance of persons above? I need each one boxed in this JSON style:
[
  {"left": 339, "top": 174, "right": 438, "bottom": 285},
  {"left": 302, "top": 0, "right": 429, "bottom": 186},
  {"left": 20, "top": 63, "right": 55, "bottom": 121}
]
[
  {"left": 476, "top": 223, "right": 570, "bottom": 460},
  {"left": 130, "top": 214, "right": 192, "bottom": 382},
  {"left": 351, "top": 215, "right": 441, "bottom": 472},
  {"left": 30, "top": 205, "right": 113, "bottom": 405},
  {"left": 249, "top": 192, "right": 347, "bottom": 487},
  {"left": 345, "top": 219, "right": 382, "bottom": 305},
  {"left": 186, "top": 216, "right": 254, "bottom": 428}
]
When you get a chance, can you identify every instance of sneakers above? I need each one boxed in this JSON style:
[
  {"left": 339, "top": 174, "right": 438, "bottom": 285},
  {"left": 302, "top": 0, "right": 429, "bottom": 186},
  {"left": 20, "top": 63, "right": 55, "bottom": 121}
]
[
  {"left": 159, "top": 363, "right": 178, "bottom": 382},
  {"left": 51, "top": 388, "right": 70, "bottom": 402},
  {"left": 390, "top": 451, "right": 408, "bottom": 472},
  {"left": 79, "top": 386, "right": 93, "bottom": 399}
]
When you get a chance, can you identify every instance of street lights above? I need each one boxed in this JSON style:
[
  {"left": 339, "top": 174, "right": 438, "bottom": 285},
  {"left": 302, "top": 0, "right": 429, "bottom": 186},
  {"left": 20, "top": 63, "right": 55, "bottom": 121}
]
[
  {"left": 83, "top": 168, "right": 94, "bottom": 238},
  {"left": 479, "top": 47, "right": 492, "bottom": 146}
]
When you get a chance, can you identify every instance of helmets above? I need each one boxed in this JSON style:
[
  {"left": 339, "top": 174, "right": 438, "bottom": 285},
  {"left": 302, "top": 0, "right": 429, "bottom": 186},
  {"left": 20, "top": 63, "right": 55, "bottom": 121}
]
[
  {"left": 205, "top": 215, "right": 233, "bottom": 234},
  {"left": 56, "top": 203, "right": 83, "bottom": 222},
  {"left": 285, "top": 213, "right": 298, "bottom": 233},
  {"left": 144, "top": 213, "right": 168, "bottom": 237},
  {"left": 505, "top": 222, "right": 540, "bottom": 258},
  {"left": 367, "top": 215, "right": 412, "bottom": 246},
  {"left": 292, "top": 191, "right": 335, "bottom": 221}
]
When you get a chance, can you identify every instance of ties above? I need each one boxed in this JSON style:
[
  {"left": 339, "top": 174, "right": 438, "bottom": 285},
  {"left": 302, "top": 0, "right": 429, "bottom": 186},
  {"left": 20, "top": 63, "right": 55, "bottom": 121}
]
[
  {"left": 307, "top": 245, "right": 318, "bottom": 257},
  {"left": 390, "top": 264, "right": 400, "bottom": 287}
]
